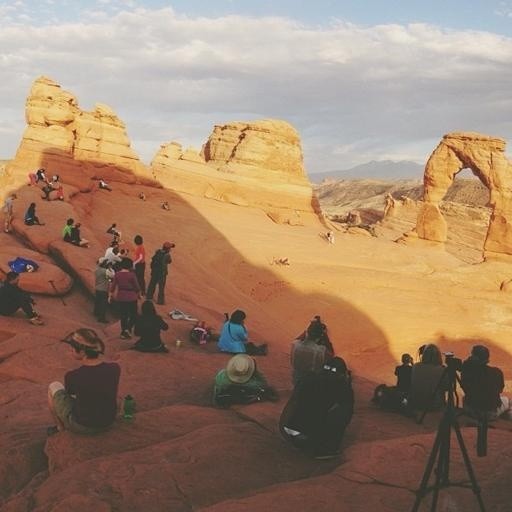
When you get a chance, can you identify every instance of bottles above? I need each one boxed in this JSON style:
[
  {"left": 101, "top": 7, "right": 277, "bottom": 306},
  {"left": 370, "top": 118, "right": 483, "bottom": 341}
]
[{"left": 123, "top": 394, "right": 135, "bottom": 418}]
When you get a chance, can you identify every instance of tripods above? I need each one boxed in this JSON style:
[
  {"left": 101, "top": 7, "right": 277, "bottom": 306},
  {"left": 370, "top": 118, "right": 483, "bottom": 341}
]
[
  {"left": 411, "top": 390, "right": 485, "bottom": 512},
  {"left": 417, "top": 367, "right": 466, "bottom": 423}
]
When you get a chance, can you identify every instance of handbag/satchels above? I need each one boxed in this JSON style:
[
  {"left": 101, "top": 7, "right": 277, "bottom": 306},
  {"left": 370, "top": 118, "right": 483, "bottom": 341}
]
[
  {"left": 169, "top": 308, "right": 208, "bottom": 344},
  {"left": 24, "top": 217, "right": 34, "bottom": 225}
]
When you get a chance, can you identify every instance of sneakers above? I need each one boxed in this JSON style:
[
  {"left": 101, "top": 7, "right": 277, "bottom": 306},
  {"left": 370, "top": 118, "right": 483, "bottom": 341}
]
[
  {"left": 47, "top": 424, "right": 59, "bottom": 436},
  {"left": 119, "top": 328, "right": 134, "bottom": 339},
  {"left": 29, "top": 310, "right": 44, "bottom": 325},
  {"left": 96, "top": 320, "right": 109, "bottom": 325}
]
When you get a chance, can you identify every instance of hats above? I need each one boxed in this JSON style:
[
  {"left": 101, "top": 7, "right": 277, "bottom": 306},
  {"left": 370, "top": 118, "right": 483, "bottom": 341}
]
[
  {"left": 60, "top": 328, "right": 105, "bottom": 353},
  {"left": 163, "top": 241, "right": 175, "bottom": 250},
  {"left": 226, "top": 353, "right": 254, "bottom": 384},
  {"left": 12, "top": 193, "right": 18, "bottom": 199},
  {"left": 97, "top": 256, "right": 108, "bottom": 266},
  {"left": 322, "top": 356, "right": 347, "bottom": 376}
]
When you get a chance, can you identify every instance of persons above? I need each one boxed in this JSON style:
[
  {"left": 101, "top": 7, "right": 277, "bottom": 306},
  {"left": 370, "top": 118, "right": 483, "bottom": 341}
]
[
  {"left": 215, "top": 308, "right": 270, "bottom": 357},
  {"left": 138, "top": 191, "right": 145, "bottom": 201},
  {"left": 277, "top": 312, "right": 512, "bottom": 462},
  {"left": 328, "top": 230, "right": 334, "bottom": 245},
  {"left": 69, "top": 222, "right": 92, "bottom": 249},
  {"left": 61, "top": 218, "right": 75, "bottom": 242},
  {"left": 0, "top": 270, "right": 46, "bottom": 327},
  {"left": 2, "top": 193, "right": 18, "bottom": 233},
  {"left": 46, "top": 328, "right": 122, "bottom": 437},
  {"left": 34, "top": 168, "right": 50, "bottom": 184},
  {"left": 160, "top": 201, "right": 171, "bottom": 210},
  {"left": 271, "top": 256, "right": 290, "bottom": 267},
  {"left": 98, "top": 177, "right": 113, "bottom": 191},
  {"left": 92, "top": 221, "right": 177, "bottom": 354},
  {"left": 40, "top": 174, "right": 60, "bottom": 200},
  {"left": 23, "top": 202, "right": 46, "bottom": 226}
]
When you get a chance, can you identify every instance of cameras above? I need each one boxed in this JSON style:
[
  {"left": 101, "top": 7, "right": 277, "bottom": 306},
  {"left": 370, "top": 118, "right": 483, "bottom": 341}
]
[{"left": 314, "top": 316, "right": 326, "bottom": 330}]
